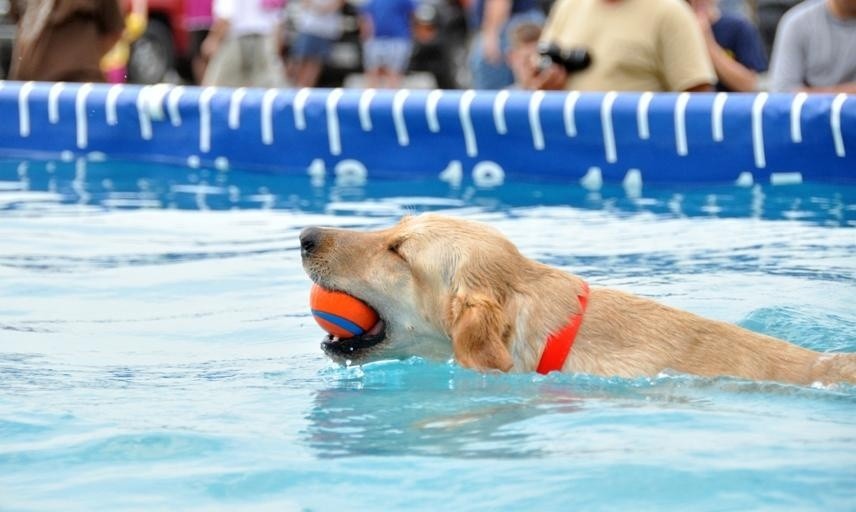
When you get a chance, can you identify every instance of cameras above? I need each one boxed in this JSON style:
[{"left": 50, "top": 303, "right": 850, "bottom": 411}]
[{"left": 535, "top": 41, "right": 592, "bottom": 73}]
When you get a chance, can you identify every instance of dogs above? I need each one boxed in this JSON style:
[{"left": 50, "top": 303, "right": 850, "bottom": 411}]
[{"left": 297, "top": 212, "right": 855, "bottom": 389}]
